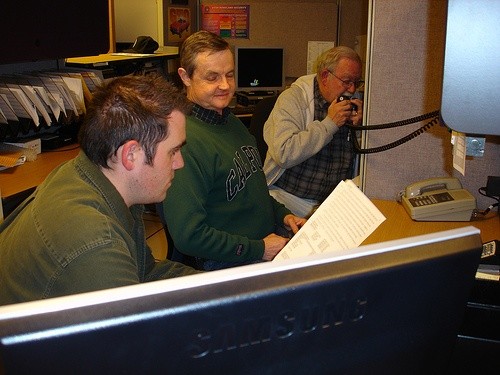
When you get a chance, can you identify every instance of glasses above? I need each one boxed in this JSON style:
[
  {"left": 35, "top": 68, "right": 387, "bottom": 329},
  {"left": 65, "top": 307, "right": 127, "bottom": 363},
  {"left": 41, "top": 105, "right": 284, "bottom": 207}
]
[{"left": 327, "top": 69, "right": 365, "bottom": 89}]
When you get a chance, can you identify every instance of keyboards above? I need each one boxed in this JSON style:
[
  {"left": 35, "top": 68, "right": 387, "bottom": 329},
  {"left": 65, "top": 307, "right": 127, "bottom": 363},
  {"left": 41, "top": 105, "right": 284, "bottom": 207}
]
[{"left": 230, "top": 106, "right": 259, "bottom": 114}]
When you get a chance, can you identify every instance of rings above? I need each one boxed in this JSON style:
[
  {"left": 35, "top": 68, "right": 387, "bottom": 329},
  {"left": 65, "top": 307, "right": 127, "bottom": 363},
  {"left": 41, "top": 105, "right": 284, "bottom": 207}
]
[{"left": 354, "top": 111, "right": 357, "bottom": 116}]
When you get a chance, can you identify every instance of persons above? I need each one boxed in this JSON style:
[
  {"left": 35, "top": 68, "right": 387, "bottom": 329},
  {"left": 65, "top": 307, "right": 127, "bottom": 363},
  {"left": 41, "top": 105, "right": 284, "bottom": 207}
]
[
  {"left": 0, "top": 73, "right": 207, "bottom": 306},
  {"left": 262, "top": 46, "right": 363, "bottom": 219},
  {"left": 156, "top": 31, "right": 307, "bottom": 272}
]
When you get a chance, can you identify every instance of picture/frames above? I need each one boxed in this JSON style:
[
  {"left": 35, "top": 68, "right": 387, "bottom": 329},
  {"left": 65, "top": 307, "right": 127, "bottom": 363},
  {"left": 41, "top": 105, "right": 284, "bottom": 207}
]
[{"left": 163, "top": 0, "right": 198, "bottom": 47}]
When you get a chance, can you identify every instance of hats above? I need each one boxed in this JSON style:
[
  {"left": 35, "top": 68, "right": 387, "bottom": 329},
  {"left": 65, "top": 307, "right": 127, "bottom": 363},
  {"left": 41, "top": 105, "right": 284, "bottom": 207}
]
[{"left": 122, "top": 36, "right": 159, "bottom": 53}]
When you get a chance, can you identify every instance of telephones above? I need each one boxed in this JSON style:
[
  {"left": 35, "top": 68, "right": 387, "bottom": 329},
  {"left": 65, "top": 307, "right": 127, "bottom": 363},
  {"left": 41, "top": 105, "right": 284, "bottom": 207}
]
[{"left": 400, "top": 177, "right": 477, "bottom": 222}]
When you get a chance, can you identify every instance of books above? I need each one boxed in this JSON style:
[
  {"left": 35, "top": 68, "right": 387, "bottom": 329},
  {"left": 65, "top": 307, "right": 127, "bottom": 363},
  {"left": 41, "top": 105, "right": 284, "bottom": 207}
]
[
  {"left": 0, "top": 68, "right": 105, "bottom": 127},
  {"left": 110, "top": 52, "right": 156, "bottom": 57}
]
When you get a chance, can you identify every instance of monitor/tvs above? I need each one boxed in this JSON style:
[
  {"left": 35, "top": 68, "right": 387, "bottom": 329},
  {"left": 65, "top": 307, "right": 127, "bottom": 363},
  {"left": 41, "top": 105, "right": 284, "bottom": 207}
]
[{"left": 234, "top": 47, "right": 285, "bottom": 92}]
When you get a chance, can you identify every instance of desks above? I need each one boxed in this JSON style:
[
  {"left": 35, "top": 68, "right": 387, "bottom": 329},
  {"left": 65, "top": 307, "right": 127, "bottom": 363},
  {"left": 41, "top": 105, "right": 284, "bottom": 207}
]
[
  {"left": 358, "top": 190, "right": 499, "bottom": 270},
  {"left": 1, "top": 45, "right": 254, "bottom": 223}
]
